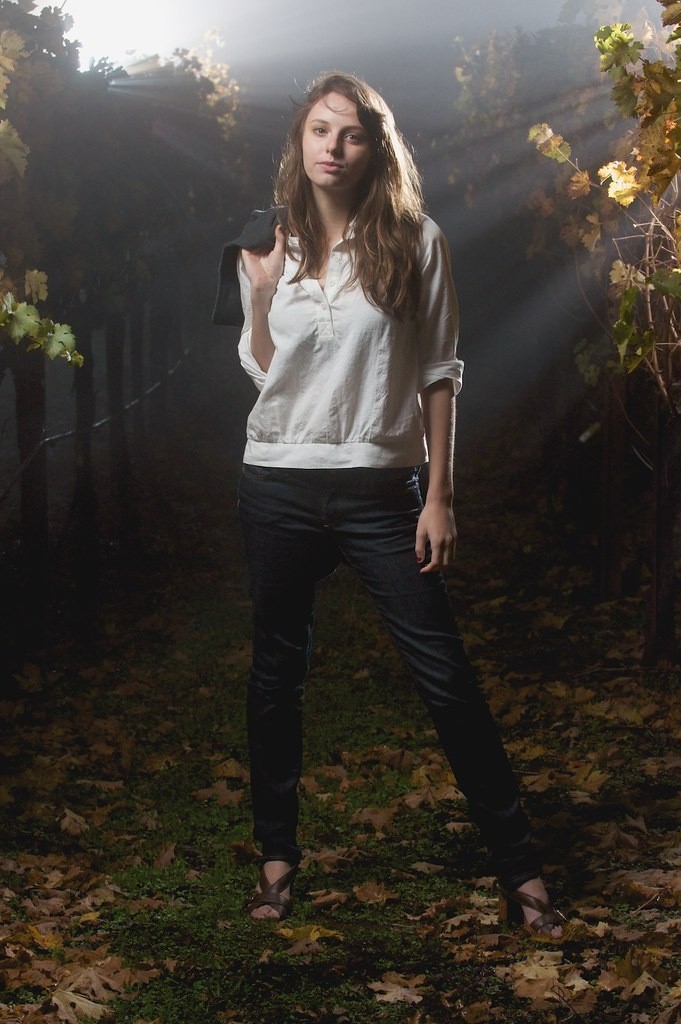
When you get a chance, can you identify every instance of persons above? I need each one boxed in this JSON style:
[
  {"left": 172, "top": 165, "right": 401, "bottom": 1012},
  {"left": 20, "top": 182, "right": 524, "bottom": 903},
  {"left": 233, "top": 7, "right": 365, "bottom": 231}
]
[{"left": 236, "top": 73, "right": 566, "bottom": 935}]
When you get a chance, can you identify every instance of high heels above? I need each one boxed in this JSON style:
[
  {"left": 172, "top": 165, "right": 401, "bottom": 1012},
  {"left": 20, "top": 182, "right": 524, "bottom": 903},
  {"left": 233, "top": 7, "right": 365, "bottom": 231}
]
[
  {"left": 498, "top": 889, "right": 570, "bottom": 943},
  {"left": 247, "top": 864, "right": 297, "bottom": 922}
]
